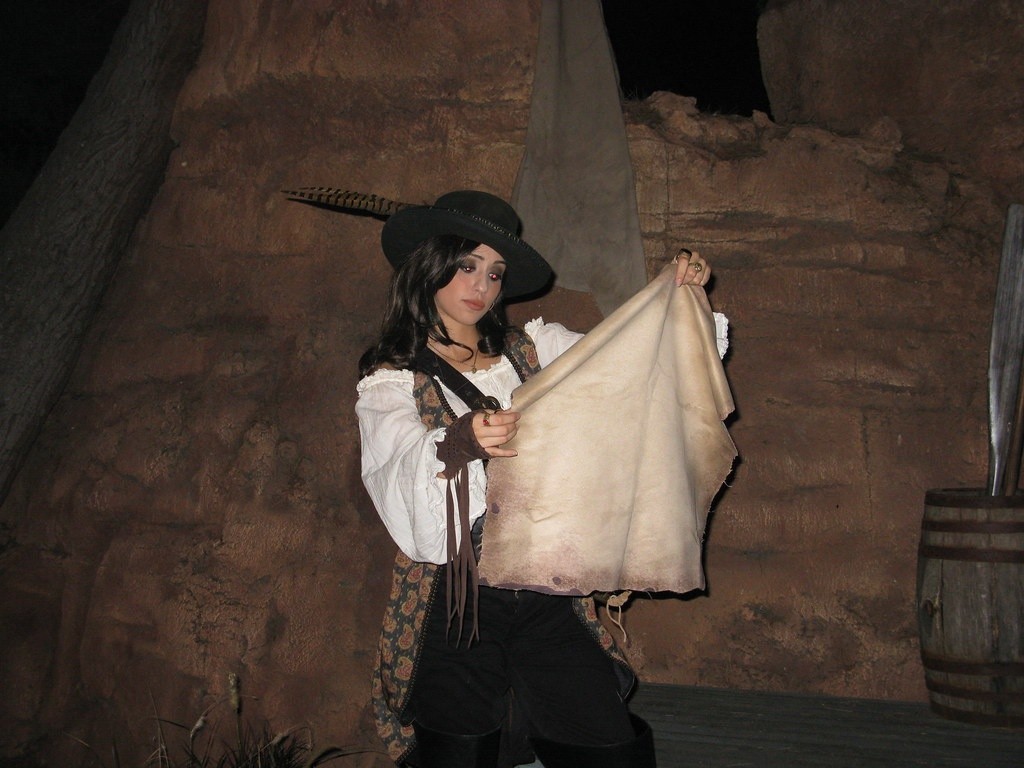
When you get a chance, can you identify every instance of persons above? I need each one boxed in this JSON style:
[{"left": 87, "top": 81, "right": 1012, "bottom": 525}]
[{"left": 355, "top": 189, "right": 729, "bottom": 768}]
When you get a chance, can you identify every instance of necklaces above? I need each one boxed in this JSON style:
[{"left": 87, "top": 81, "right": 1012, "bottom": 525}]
[{"left": 428, "top": 342, "right": 479, "bottom": 374}]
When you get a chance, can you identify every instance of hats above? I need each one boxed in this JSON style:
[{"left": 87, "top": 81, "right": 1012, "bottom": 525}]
[{"left": 381, "top": 189, "right": 554, "bottom": 300}]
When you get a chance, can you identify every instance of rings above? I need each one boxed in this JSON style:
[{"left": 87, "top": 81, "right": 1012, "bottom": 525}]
[
  {"left": 688, "top": 262, "right": 702, "bottom": 272},
  {"left": 483, "top": 414, "right": 491, "bottom": 426},
  {"left": 679, "top": 248, "right": 692, "bottom": 261}
]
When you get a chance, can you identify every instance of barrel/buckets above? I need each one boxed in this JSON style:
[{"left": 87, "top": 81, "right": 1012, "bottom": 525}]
[{"left": 918, "top": 488, "right": 1024, "bottom": 728}]
